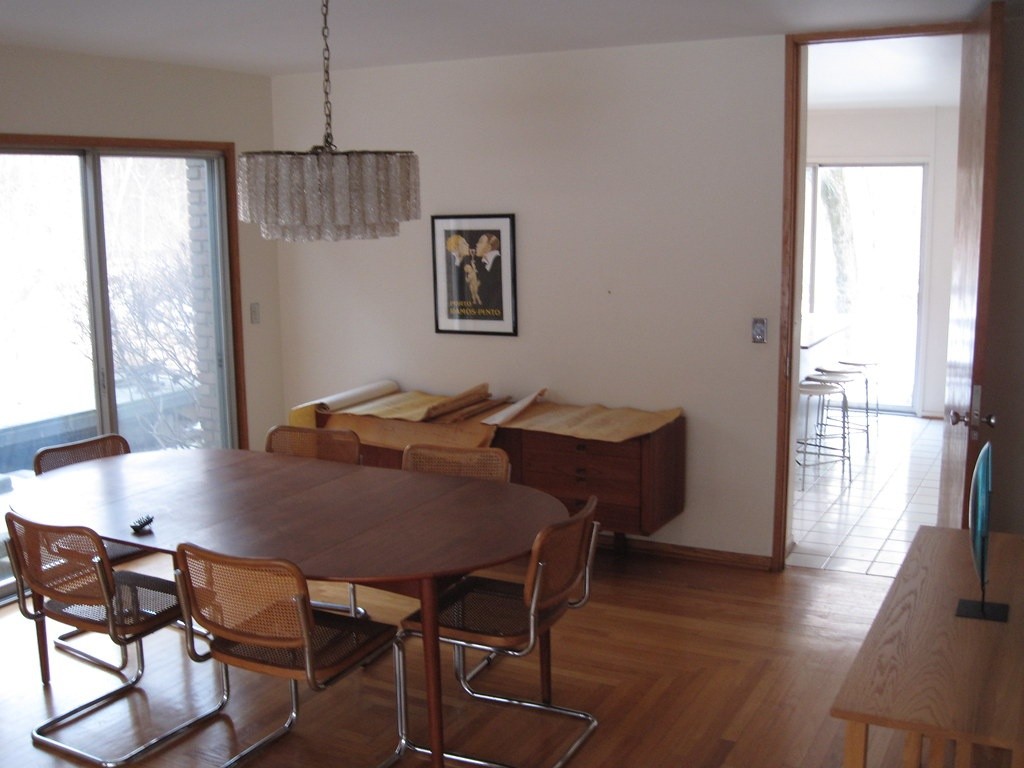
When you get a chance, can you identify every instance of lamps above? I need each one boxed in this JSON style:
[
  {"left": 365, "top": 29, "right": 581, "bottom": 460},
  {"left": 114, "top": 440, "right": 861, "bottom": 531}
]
[
  {"left": 431, "top": 213, "right": 518, "bottom": 335},
  {"left": 233, "top": 2, "right": 421, "bottom": 244}
]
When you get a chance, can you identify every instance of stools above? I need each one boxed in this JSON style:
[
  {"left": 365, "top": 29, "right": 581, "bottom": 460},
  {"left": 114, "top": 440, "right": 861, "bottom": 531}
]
[{"left": 797, "top": 354, "right": 889, "bottom": 486}]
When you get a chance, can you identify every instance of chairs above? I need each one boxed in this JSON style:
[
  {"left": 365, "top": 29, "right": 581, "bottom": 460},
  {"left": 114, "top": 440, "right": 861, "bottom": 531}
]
[{"left": 4, "top": 424, "right": 602, "bottom": 767}]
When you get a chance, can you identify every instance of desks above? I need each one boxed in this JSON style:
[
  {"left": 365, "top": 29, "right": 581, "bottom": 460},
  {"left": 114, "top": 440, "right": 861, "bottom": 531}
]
[
  {"left": 14, "top": 446, "right": 572, "bottom": 767},
  {"left": 828, "top": 524, "right": 1024, "bottom": 768}
]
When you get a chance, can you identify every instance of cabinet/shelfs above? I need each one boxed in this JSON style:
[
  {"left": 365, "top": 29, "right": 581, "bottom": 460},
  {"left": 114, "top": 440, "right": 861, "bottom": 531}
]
[{"left": 314, "top": 404, "right": 687, "bottom": 555}]
